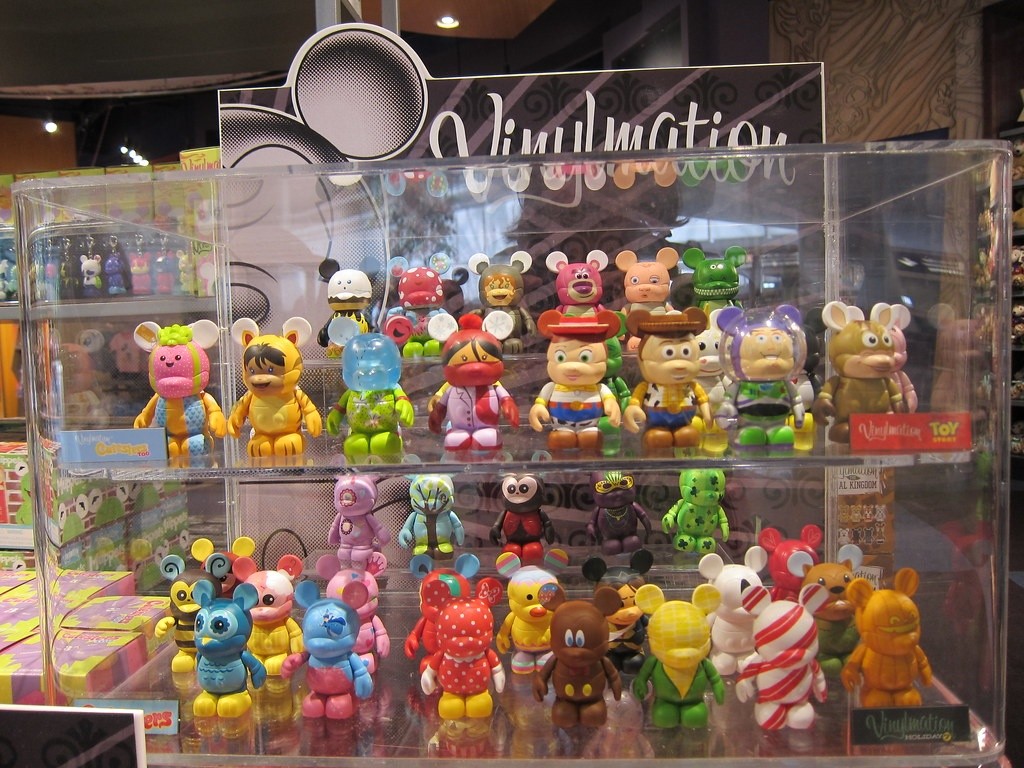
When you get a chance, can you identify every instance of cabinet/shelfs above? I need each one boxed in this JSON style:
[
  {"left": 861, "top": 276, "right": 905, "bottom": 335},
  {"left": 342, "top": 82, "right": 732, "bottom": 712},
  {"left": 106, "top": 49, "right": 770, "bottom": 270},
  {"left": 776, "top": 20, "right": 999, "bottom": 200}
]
[
  {"left": 12, "top": 138, "right": 1015, "bottom": 767},
  {"left": 981, "top": 0, "right": 1024, "bottom": 491}
]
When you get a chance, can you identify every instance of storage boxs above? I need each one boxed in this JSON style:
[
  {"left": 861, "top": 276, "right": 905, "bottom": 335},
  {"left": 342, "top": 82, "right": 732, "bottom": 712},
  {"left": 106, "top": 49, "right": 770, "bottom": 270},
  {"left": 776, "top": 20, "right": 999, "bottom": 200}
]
[
  {"left": 1, "top": 146, "right": 221, "bottom": 297},
  {"left": 0, "top": 441, "right": 190, "bottom": 592},
  {"left": 0, "top": 567, "right": 175, "bottom": 707}
]
